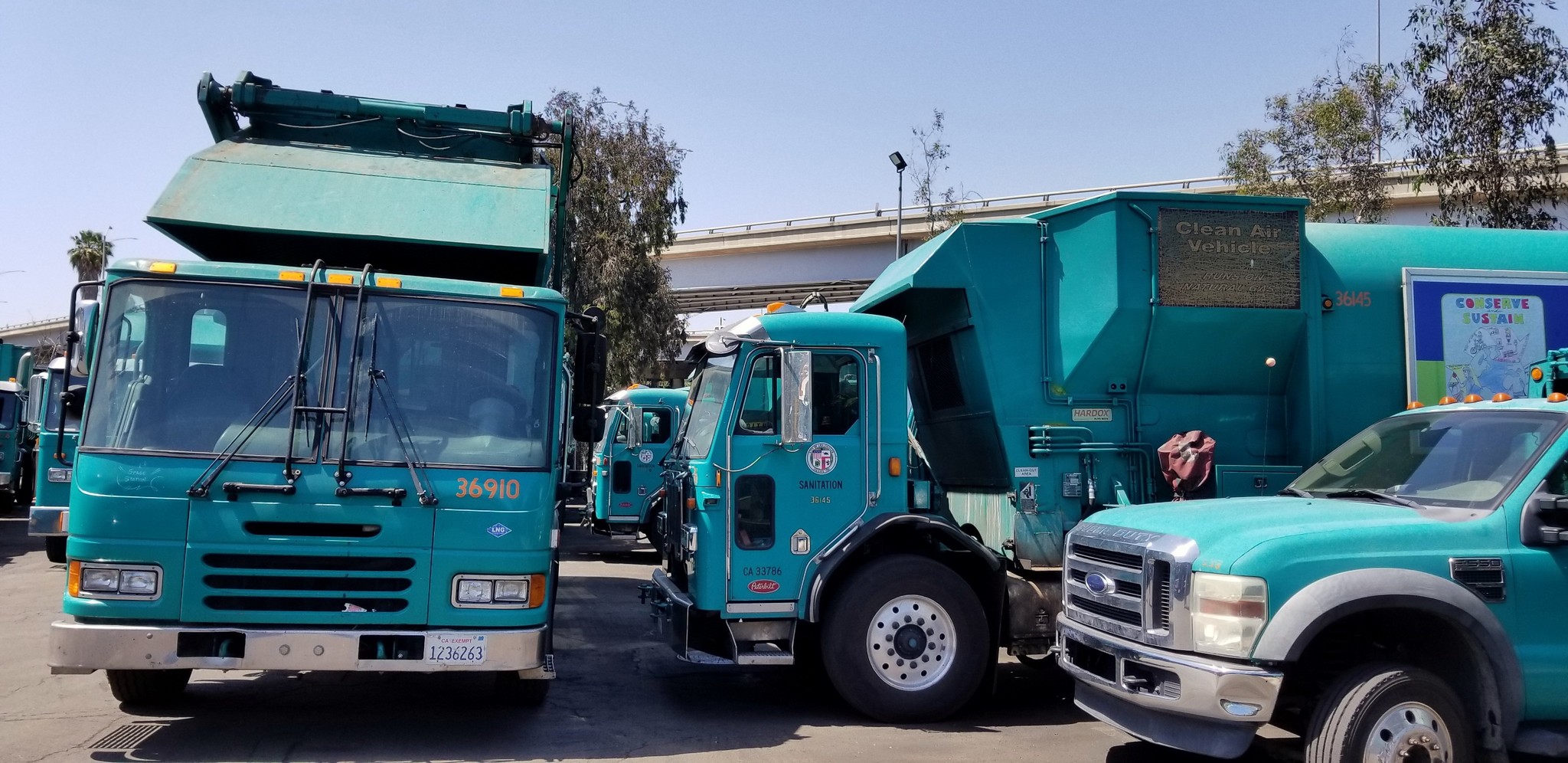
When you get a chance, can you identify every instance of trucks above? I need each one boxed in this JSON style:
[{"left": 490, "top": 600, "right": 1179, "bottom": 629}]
[
  {"left": 648, "top": 189, "right": 1568, "bottom": 723},
  {"left": 582, "top": 385, "right": 692, "bottom": 560},
  {"left": 45, "top": 69, "right": 572, "bottom": 708},
  {"left": 0, "top": 343, "right": 143, "bottom": 563},
  {"left": 1052, "top": 346, "right": 1568, "bottom": 763}
]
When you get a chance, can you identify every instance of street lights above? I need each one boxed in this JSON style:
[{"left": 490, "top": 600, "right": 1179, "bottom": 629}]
[{"left": 888, "top": 151, "right": 907, "bottom": 259}]
